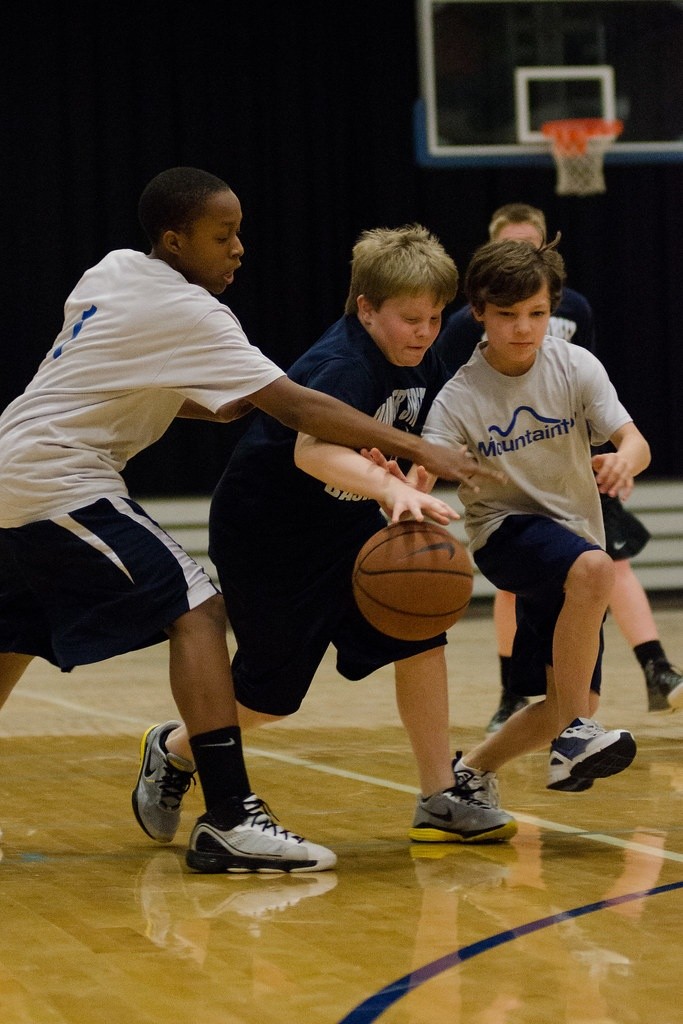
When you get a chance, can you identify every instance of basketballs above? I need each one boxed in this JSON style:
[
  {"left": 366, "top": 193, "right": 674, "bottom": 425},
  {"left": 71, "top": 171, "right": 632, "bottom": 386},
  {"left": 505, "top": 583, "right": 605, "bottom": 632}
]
[{"left": 352, "top": 520, "right": 474, "bottom": 642}]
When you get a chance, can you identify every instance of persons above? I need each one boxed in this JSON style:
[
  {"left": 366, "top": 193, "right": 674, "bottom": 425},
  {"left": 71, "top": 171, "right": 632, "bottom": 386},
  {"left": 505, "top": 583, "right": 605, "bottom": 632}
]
[
  {"left": 134, "top": 762, "right": 683, "bottom": 1024},
  {"left": 0, "top": 166, "right": 683, "bottom": 873}
]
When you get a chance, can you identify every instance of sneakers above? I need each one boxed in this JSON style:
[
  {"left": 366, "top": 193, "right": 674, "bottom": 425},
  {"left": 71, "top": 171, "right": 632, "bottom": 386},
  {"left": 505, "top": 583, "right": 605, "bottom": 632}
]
[
  {"left": 451, "top": 750, "right": 499, "bottom": 811},
  {"left": 407, "top": 770, "right": 515, "bottom": 841},
  {"left": 196, "top": 872, "right": 338, "bottom": 920},
  {"left": 546, "top": 720, "right": 637, "bottom": 792},
  {"left": 410, "top": 844, "right": 517, "bottom": 894},
  {"left": 641, "top": 661, "right": 683, "bottom": 715},
  {"left": 484, "top": 689, "right": 528, "bottom": 735},
  {"left": 187, "top": 792, "right": 336, "bottom": 874},
  {"left": 135, "top": 855, "right": 207, "bottom": 965},
  {"left": 132, "top": 721, "right": 200, "bottom": 845}
]
